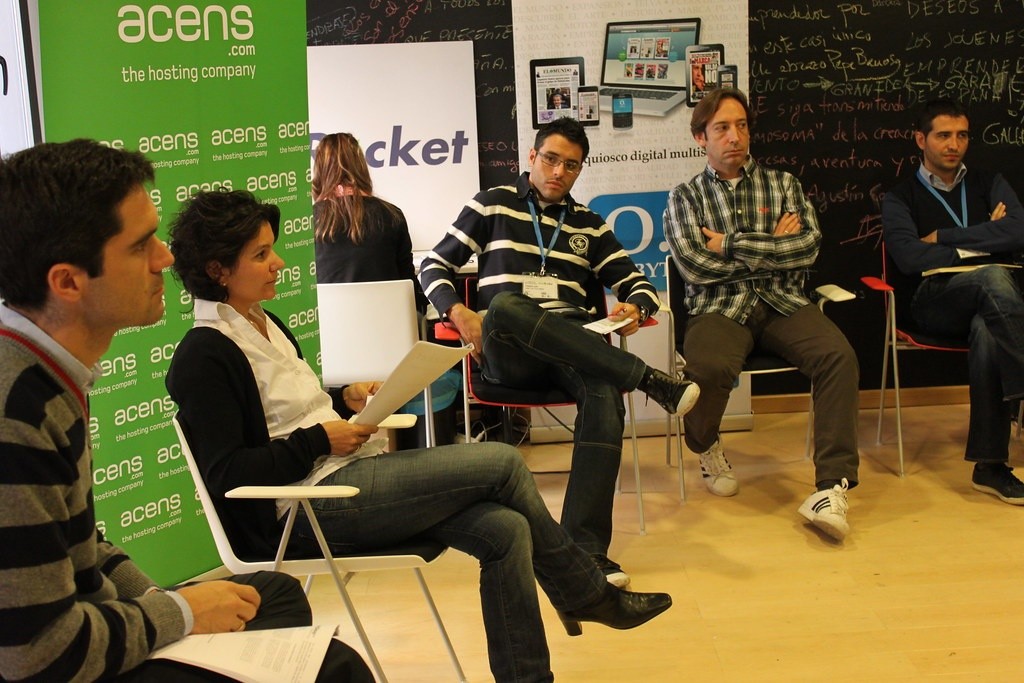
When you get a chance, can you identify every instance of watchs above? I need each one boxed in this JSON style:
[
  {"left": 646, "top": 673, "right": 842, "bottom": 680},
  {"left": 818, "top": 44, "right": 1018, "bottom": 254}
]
[{"left": 638, "top": 304, "right": 649, "bottom": 326}]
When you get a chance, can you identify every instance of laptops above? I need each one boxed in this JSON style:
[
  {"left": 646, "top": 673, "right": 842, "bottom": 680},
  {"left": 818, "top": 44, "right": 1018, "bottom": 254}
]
[{"left": 595, "top": 17, "right": 701, "bottom": 117}]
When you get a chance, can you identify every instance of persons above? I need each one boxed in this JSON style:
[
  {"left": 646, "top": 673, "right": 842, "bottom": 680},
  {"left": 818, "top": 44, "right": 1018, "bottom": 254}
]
[
  {"left": 879, "top": 98, "right": 1024, "bottom": 506},
  {"left": 421, "top": 118, "right": 701, "bottom": 591},
  {"left": 165, "top": 185, "right": 673, "bottom": 683},
  {"left": 663, "top": 87, "right": 861, "bottom": 542},
  {"left": 549, "top": 94, "right": 569, "bottom": 109},
  {"left": 312, "top": 132, "right": 430, "bottom": 315},
  {"left": 692, "top": 64, "right": 704, "bottom": 92},
  {"left": 0, "top": 137, "right": 372, "bottom": 683}
]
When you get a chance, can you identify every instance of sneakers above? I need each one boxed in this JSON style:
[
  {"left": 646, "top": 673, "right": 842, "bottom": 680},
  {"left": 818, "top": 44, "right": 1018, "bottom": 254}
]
[
  {"left": 796, "top": 487, "right": 848, "bottom": 541},
  {"left": 700, "top": 434, "right": 739, "bottom": 496},
  {"left": 640, "top": 369, "right": 700, "bottom": 418},
  {"left": 589, "top": 555, "right": 632, "bottom": 587},
  {"left": 971, "top": 463, "right": 1024, "bottom": 505}
]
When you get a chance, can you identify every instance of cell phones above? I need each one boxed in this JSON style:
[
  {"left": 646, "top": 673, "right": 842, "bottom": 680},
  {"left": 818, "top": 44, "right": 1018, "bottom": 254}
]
[
  {"left": 578, "top": 86, "right": 599, "bottom": 126},
  {"left": 717, "top": 65, "right": 738, "bottom": 88},
  {"left": 612, "top": 92, "right": 633, "bottom": 131}
]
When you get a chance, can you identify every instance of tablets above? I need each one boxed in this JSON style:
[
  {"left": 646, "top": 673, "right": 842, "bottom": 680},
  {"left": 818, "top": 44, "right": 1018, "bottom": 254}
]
[
  {"left": 685, "top": 43, "right": 724, "bottom": 109},
  {"left": 530, "top": 57, "right": 585, "bottom": 130}
]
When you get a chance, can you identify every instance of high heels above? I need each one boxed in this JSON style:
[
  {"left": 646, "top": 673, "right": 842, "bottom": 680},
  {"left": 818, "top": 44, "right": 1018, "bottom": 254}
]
[{"left": 557, "top": 585, "right": 673, "bottom": 636}]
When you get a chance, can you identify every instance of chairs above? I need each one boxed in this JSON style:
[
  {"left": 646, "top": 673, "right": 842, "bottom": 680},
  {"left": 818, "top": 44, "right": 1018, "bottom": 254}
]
[
  {"left": 315, "top": 279, "right": 439, "bottom": 449},
  {"left": 861, "top": 238, "right": 1024, "bottom": 480},
  {"left": 432, "top": 274, "right": 661, "bottom": 537},
  {"left": 174, "top": 409, "right": 472, "bottom": 683},
  {"left": 666, "top": 253, "right": 857, "bottom": 503}
]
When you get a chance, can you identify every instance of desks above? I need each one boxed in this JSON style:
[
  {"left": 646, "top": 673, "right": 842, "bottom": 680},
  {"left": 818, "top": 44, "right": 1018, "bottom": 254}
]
[{"left": 409, "top": 247, "right": 483, "bottom": 277}]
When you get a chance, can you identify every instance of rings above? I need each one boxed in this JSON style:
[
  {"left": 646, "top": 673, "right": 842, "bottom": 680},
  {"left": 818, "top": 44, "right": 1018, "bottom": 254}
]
[
  {"left": 237, "top": 620, "right": 244, "bottom": 632},
  {"left": 354, "top": 445, "right": 359, "bottom": 453}
]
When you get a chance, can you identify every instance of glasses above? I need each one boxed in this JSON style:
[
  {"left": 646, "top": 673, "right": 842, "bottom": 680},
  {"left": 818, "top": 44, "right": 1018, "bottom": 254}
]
[{"left": 535, "top": 150, "right": 582, "bottom": 175}]
